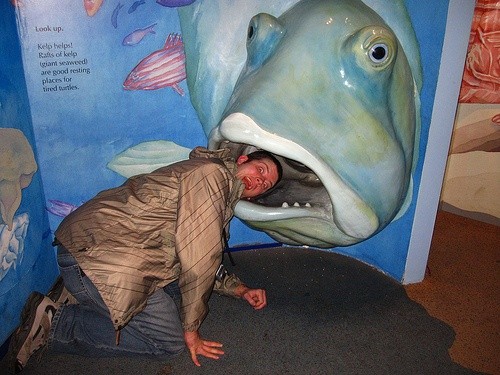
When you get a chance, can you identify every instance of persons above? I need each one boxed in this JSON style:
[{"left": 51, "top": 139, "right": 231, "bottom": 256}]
[{"left": 7, "top": 146, "right": 283, "bottom": 368}]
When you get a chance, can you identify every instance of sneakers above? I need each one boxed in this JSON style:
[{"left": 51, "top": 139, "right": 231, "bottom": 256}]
[
  {"left": 47, "top": 276, "right": 79, "bottom": 307},
  {"left": 8, "top": 291, "right": 58, "bottom": 374}
]
